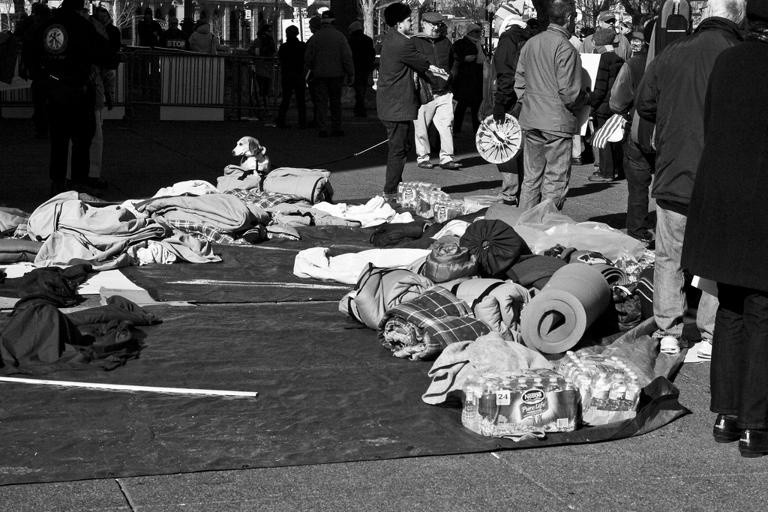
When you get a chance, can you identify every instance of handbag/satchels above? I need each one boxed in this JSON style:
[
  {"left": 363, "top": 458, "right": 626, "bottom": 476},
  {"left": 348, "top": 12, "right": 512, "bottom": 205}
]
[{"left": 419, "top": 77, "right": 434, "bottom": 104}]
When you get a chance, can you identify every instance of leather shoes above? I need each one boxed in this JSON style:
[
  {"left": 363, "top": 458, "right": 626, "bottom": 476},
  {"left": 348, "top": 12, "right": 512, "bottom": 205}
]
[{"left": 714, "top": 415, "right": 768, "bottom": 457}]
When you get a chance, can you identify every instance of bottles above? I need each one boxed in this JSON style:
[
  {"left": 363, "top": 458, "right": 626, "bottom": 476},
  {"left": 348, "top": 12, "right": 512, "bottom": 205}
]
[
  {"left": 397, "top": 181, "right": 466, "bottom": 225},
  {"left": 460, "top": 370, "right": 578, "bottom": 437},
  {"left": 562, "top": 351, "right": 642, "bottom": 428}
]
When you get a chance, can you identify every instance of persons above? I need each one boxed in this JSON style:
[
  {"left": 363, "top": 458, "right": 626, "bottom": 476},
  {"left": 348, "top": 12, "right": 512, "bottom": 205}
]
[
  {"left": 303, "top": 16, "right": 322, "bottom": 127},
  {"left": 493, "top": 5, "right": 528, "bottom": 205},
  {"left": 453, "top": 24, "right": 487, "bottom": 132},
  {"left": 138, "top": 8, "right": 163, "bottom": 97},
  {"left": 179, "top": 19, "right": 220, "bottom": 54},
  {"left": 527, "top": 18, "right": 539, "bottom": 35},
  {"left": 514, "top": 0, "right": 582, "bottom": 211},
  {"left": 252, "top": 25, "right": 275, "bottom": 116},
  {"left": 279, "top": 26, "right": 307, "bottom": 124},
  {"left": 376, "top": 0, "right": 448, "bottom": 208},
  {"left": 569, "top": 0, "right": 693, "bottom": 241},
  {"left": 305, "top": 11, "right": 354, "bottom": 137},
  {"left": 160, "top": 18, "right": 187, "bottom": 49},
  {"left": 410, "top": 12, "right": 462, "bottom": 168},
  {"left": 636, "top": 0, "right": 746, "bottom": 359},
  {"left": 350, "top": 22, "right": 374, "bottom": 118},
  {"left": 683, "top": 0, "right": 768, "bottom": 458},
  {"left": 0, "top": 0, "right": 122, "bottom": 193}
]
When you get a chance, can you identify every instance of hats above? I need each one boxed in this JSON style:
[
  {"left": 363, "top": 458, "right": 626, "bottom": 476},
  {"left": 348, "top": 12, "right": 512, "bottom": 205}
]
[
  {"left": 496, "top": 4, "right": 521, "bottom": 25},
  {"left": 422, "top": 12, "right": 442, "bottom": 23},
  {"left": 599, "top": 11, "right": 615, "bottom": 21}
]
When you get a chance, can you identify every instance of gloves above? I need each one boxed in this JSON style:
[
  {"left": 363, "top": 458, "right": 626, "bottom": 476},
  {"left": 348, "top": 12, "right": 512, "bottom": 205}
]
[{"left": 494, "top": 104, "right": 504, "bottom": 120}]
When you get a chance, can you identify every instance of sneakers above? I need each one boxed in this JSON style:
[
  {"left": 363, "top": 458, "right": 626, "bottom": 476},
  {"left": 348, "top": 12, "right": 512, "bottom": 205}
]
[
  {"left": 440, "top": 162, "right": 462, "bottom": 168},
  {"left": 660, "top": 336, "right": 688, "bottom": 353},
  {"left": 419, "top": 162, "right": 432, "bottom": 168},
  {"left": 697, "top": 341, "right": 712, "bottom": 358},
  {"left": 589, "top": 172, "right": 619, "bottom": 181}
]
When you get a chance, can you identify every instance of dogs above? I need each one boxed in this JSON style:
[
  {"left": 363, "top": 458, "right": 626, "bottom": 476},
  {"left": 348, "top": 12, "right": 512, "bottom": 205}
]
[{"left": 230, "top": 136, "right": 272, "bottom": 174}]
